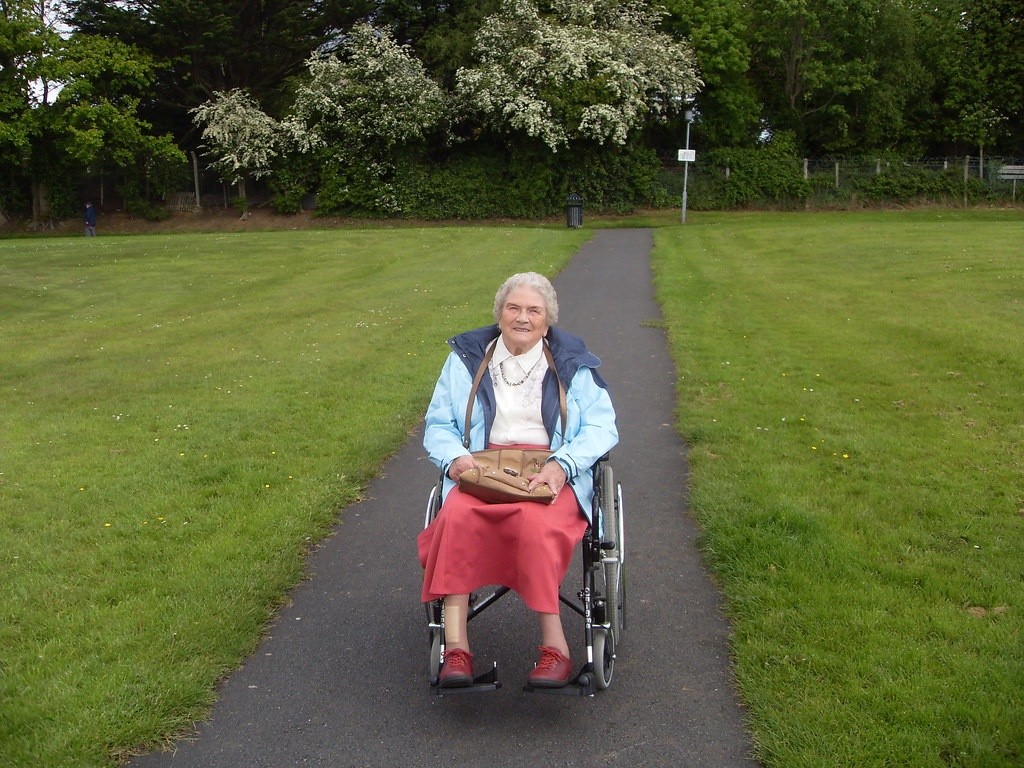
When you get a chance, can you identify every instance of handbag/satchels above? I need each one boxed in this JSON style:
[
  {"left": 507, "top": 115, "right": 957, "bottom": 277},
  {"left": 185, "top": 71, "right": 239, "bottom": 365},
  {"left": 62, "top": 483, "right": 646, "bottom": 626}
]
[{"left": 459, "top": 448, "right": 554, "bottom": 505}]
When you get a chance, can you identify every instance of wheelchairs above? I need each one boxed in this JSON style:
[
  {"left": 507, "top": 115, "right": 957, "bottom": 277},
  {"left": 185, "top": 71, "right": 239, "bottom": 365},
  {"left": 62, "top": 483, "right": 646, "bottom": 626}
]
[{"left": 422, "top": 448, "right": 625, "bottom": 697}]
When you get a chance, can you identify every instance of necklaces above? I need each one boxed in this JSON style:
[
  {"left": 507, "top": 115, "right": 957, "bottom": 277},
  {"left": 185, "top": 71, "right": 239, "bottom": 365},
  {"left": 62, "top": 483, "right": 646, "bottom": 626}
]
[{"left": 499, "top": 350, "right": 543, "bottom": 386}]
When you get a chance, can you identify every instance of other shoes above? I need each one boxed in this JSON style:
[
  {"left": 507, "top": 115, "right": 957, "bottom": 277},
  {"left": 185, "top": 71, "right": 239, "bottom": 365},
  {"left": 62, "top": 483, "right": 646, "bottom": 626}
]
[
  {"left": 438, "top": 648, "right": 474, "bottom": 688},
  {"left": 527, "top": 645, "right": 580, "bottom": 688}
]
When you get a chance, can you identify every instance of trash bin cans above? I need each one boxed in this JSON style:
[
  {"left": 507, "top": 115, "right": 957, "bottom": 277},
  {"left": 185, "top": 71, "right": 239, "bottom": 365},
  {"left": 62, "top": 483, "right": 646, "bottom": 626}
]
[{"left": 565, "top": 192, "right": 583, "bottom": 227}]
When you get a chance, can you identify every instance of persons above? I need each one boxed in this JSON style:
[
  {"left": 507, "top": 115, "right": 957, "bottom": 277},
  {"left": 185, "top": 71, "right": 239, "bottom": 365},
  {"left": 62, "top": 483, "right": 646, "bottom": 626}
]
[{"left": 416, "top": 272, "right": 620, "bottom": 689}]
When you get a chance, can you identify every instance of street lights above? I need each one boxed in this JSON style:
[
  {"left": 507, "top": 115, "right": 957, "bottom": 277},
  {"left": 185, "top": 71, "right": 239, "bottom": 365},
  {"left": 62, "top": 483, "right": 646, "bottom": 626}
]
[{"left": 680, "top": 105, "right": 695, "bottom": 224}]
